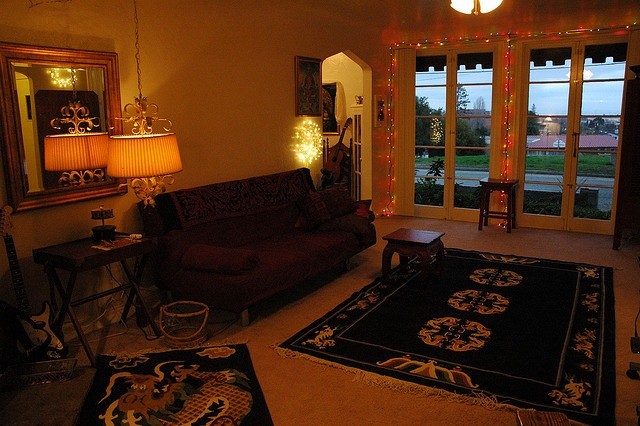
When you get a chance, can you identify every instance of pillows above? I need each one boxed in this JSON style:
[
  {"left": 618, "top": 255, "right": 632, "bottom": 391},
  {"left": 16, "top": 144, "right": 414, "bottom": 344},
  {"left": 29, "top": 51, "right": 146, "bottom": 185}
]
[
  {"left": 298, "top": 183, "right": 357, "bottom": 225},
  {"left": 323, "top": 199, "right": 373, "bottom": 238}
]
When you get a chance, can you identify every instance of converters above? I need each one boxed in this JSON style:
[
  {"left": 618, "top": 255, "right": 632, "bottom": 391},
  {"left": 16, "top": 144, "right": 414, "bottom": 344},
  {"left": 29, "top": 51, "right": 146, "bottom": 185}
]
[{"left": 631, "top": 337, "right": 640, "bottom": 353}]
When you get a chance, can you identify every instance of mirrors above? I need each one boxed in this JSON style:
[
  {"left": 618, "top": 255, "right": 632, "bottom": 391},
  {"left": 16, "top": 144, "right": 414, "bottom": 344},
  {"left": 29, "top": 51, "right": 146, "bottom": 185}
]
[{"left": 0, "top": 38, "right": 129, "bottom": 215}]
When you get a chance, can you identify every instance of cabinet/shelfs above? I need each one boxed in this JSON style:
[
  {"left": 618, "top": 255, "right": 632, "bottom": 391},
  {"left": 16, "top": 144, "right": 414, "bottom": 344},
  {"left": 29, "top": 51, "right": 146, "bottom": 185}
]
[
  {"left": 350, "top": 104, "right": 364, "bottom": 203},
  {"left": 610, "top": 79, "right": 640, "bottom": 253}
]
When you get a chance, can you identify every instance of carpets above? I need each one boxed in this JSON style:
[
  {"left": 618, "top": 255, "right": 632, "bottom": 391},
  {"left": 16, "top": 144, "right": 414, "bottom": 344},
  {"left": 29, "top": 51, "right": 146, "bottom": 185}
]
[
  {"left": 270, "top": 246, "right": 618, "bottom": 425},
  {"left": 73, "top": 343, "right": 274, "bottom": 426}
]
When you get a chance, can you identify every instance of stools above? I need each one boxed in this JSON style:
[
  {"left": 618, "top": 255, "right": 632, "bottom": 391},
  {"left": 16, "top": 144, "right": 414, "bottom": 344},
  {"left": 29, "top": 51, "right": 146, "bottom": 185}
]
[
  {"left": 477, "top": 176, "right": 519, "bottom": 234},
  {"left": 381, "top": 227, "right": 445, "bottom": 283}
]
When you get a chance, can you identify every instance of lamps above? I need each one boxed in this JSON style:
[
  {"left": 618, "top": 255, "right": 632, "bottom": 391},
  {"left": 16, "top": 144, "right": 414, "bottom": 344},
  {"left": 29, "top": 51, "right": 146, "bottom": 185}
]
[
  {"left": 447, "top": 0, "right": 506, "bottom": 17},
  {"left": 104, "top": 0, "right": 183, "bottom": 212},
  {"left": 44, "top": 65, "right": 110, "bottom": 184}
]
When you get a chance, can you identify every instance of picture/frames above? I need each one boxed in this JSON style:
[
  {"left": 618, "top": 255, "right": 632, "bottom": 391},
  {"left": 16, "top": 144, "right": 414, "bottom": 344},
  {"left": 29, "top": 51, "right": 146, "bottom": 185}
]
[
  {"left": 293, "top": 55, "right": 322, "bottom": 118},
  {"left": 323, "top": 81, "right": 341, "bottom": 136}
]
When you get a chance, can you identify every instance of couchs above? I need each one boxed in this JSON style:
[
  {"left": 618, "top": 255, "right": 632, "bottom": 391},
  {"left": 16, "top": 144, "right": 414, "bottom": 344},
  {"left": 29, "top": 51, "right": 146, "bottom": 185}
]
[{"left": 135, "top": 168, "right": 377, "bottom": 326}]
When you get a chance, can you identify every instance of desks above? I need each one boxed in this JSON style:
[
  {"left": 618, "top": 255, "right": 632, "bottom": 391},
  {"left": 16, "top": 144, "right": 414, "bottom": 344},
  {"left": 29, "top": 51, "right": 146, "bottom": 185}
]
[{"left": 32, "top": 232, "right": 163, "bottom": 369}]
